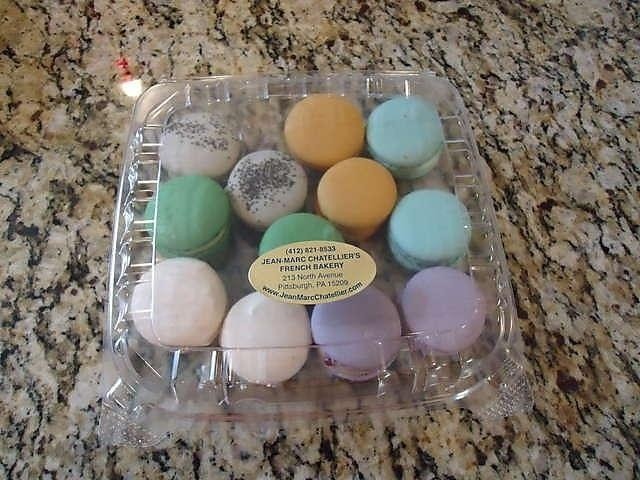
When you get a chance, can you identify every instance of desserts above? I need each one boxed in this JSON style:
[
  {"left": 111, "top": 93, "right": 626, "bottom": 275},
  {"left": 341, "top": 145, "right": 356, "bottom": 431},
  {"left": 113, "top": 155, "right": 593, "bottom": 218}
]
[{"left": 131, "top": 90, "right": 487, "bottom": 385}]
